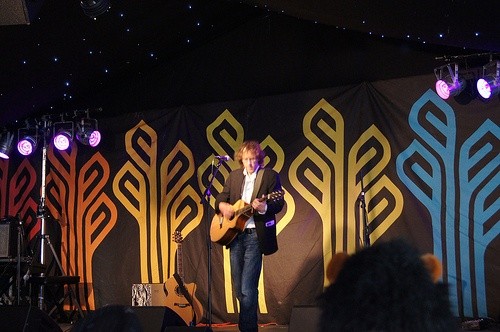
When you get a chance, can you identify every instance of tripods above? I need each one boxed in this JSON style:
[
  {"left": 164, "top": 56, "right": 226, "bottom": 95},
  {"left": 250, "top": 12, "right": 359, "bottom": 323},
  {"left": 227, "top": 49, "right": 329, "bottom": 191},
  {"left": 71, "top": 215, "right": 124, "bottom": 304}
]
[{"left": 22, "top": 146, "right": 83, "bottom": 317}]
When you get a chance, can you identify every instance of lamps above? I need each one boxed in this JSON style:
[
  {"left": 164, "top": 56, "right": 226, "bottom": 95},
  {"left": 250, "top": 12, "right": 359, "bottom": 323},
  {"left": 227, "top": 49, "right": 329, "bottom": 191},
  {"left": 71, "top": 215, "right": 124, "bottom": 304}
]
[
  {"left": 16, "top": 126, "right": 40, "bottom": 156},
  {"left": 0, "top": 128, "right": 17, "bottom": 159},
  {"left": 475, "top": 59, "right": 500, "bottom": 102},
  {"left": 75, "top": 118, "right": 101, "bottom": 147},
  {"left": 433, "top": 63, "right": 467, "bottom": 100},
  {"left": 53, "top": 121, "right": 75, "bottom": 150}
]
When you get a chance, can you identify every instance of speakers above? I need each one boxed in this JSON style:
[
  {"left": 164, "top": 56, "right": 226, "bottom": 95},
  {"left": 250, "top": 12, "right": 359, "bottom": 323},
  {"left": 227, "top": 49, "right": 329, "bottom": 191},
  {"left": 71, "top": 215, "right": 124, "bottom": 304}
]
[
  {"left": 288, "top": 305, "right": 323, "bottom": 332},
  {"left": 0, "top": 224, "right": 11, "bottom": 257},
  {"left": 68, "top": 305, "right": 212, "bottom": 332}
]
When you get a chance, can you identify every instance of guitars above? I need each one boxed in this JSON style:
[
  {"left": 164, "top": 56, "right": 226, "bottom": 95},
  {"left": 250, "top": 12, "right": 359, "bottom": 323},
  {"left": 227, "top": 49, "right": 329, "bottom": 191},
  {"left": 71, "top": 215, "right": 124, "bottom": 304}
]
[{"left": 209, "top": 186, "right": 285, "bottom": 246}]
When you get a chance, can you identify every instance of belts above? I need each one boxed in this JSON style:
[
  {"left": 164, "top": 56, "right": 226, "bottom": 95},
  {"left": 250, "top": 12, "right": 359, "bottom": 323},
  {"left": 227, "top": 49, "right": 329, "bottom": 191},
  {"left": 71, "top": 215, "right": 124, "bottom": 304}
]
[{"left": 244, "top": 229, "right": 257, "bottom": 234}]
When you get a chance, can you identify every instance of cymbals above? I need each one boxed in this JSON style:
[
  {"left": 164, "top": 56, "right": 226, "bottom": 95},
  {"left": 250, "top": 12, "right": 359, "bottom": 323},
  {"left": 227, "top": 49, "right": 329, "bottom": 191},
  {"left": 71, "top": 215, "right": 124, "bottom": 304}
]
[{"left": 159, "top": 229, "right": 205, "bottom": 327}]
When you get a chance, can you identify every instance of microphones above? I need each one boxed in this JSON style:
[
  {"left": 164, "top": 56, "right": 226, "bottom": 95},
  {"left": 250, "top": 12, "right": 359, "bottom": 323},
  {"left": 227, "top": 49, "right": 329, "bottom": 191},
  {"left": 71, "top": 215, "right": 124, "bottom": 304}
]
[{"left": 215, "top": 154, "right": 230, "bottom": 161}]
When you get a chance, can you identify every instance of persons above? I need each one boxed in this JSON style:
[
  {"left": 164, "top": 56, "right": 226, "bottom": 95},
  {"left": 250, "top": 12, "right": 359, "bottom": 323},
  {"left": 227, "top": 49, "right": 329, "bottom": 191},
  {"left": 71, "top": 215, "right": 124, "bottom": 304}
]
[
  {"left": 315, "top": 240, "right": 465, "bottom": 332},
  {"left": 215, "top": 142, "right": 285, "bottom": 332}
]
